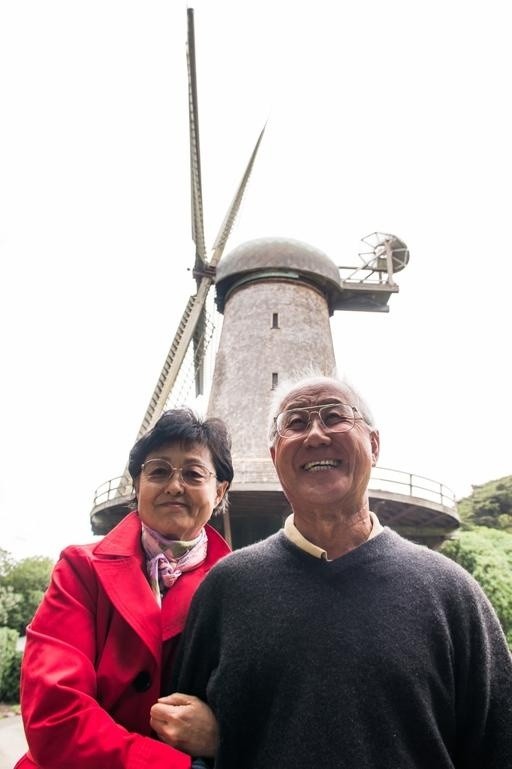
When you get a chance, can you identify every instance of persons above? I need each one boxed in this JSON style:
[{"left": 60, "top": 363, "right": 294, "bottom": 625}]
[
  {"left": 15, "top": 407, "right": 234, "bottom": 769},
  {"left": 154, "top": 379, "right": 512, "bottom": 769}
]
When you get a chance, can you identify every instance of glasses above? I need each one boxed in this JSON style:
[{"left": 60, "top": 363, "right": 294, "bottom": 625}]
[
  {"left": 271, "top": 401, "right": 365, "bottom": 440},
  {"left": 140, "top": 457, "right": 221, "bottom": 489}
]
